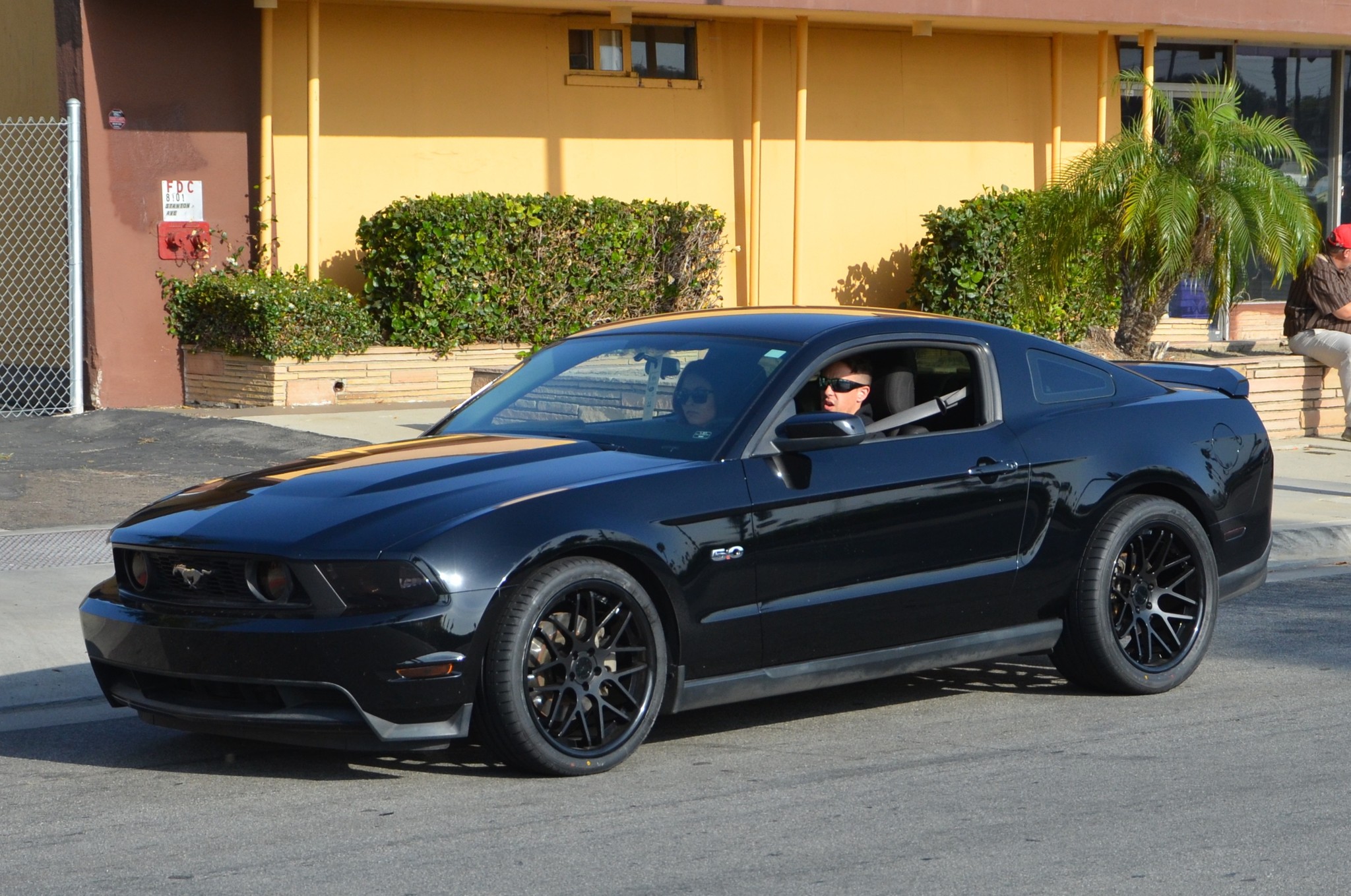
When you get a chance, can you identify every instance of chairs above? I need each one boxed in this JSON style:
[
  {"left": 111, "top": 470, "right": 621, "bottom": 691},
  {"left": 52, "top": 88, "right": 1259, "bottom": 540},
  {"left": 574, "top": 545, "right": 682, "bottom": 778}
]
[{"left": 869, "top": 355, "right": 920, "bottom": 438}]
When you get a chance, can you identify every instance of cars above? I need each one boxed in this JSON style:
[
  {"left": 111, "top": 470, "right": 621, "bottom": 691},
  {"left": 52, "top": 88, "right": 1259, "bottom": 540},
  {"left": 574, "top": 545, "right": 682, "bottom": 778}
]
[{"left": 78, "top": 304, "right": 1275, "bottom": 776}]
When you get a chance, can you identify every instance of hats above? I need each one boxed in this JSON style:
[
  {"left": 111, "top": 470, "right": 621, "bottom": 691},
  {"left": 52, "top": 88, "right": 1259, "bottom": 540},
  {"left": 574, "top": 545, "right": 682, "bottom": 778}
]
[{"left": 1329, "top": 224, "right": 1351, "bottom": 249}]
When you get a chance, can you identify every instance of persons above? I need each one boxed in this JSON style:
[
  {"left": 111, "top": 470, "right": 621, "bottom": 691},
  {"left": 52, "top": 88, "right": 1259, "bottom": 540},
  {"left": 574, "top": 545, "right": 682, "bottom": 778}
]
[
  {"left": 819, "top": 353, "right": 886, "bottom": 440},
  {"left": 1283, "top": 224, "right": 1351, "bottom": 441},
  {"left": 671, "top": 358, "right": 738, "bottom": 431}
]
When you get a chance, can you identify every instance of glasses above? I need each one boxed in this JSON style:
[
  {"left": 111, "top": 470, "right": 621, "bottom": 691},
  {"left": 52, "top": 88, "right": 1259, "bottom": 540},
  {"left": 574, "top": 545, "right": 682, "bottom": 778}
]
[
  {"left": 818, "top": 376, "right": 869, "bottom": 393},
  {"left": 675, "top": 389, "right": 713, "bottom": 404}
]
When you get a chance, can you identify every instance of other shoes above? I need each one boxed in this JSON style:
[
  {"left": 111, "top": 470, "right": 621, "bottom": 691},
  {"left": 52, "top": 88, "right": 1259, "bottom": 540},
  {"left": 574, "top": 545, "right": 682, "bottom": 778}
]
[{"left": 1340, "top": 428, "right": 1351, "bottom": 441}]
